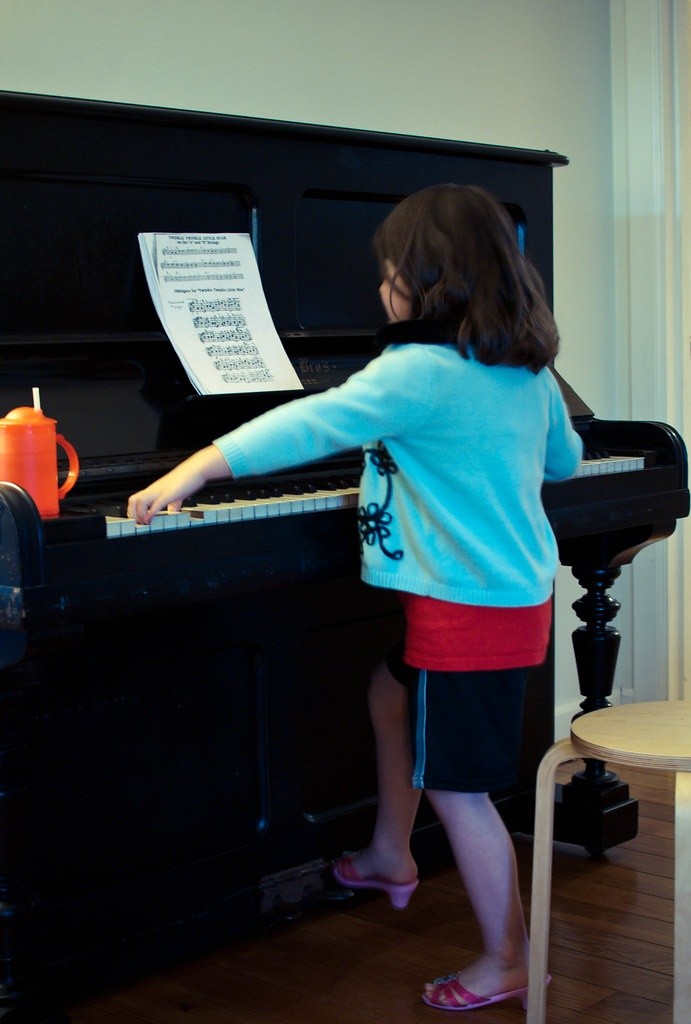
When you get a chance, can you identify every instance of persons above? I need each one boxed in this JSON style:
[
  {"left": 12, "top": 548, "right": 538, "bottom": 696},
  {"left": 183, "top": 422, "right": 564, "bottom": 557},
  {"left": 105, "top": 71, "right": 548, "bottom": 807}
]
[{"left": 126, "top": 183, "right": 583, "bottom": 1009}]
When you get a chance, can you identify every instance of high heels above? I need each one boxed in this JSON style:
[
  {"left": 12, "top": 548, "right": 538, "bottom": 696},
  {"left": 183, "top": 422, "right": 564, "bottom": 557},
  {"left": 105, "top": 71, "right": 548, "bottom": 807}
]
[
  {"left": 422, "top": 970, "right": 552, "bottom": 1010},
  {"left": 333, "top": 851, "right": 420, "bottom": 909}
]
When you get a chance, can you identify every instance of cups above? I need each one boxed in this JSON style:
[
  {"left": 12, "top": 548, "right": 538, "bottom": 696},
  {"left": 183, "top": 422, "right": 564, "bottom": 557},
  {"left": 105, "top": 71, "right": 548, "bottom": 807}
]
[{"left": 0, "top": 407, "right": 80, "bottom": 520}]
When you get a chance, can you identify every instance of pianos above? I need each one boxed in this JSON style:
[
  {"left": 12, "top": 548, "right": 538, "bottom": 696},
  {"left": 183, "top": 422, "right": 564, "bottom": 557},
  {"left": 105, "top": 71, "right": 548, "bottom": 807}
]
[{"left": 0, "top": 87, "right": 691, "bottom": 1024}]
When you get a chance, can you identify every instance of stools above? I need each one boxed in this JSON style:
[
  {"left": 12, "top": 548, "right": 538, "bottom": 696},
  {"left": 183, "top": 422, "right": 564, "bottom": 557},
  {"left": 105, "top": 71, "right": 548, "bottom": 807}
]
[{"left": 527, "top": 700, "right": 691, "bottom": 1024}]
